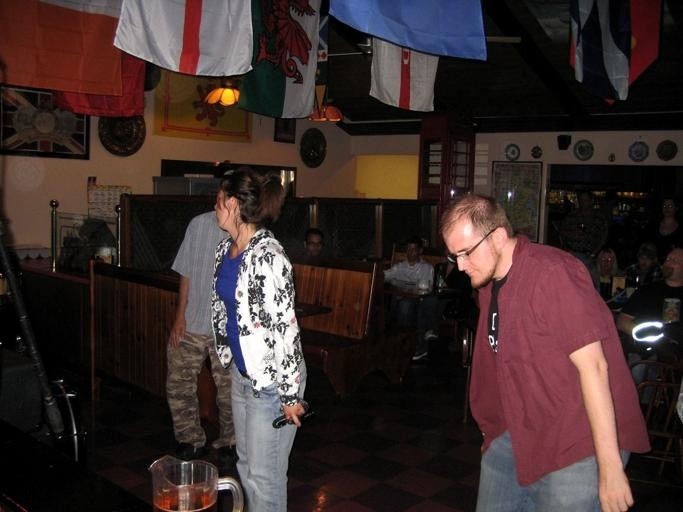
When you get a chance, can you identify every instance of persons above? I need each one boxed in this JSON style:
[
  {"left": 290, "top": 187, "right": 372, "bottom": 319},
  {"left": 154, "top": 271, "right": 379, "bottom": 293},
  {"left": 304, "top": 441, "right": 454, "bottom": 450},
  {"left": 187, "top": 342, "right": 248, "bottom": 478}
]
[
  {"left": 382, "top": 239, "right": 434, "bottom": 316},
  {"left": 409, "top": 258, "right": 471, "bottom": 361},
  {"left": 438, "top": 193, "right": 653, "bottom": 512},
  {"left": 211, "top": 170, "right": 310, "bottom": 512},
  {"left": 166, "top": 211, "right": 237, "bottom": 450},
  {"left": 615, "top": 248, "right": 683, "bottom": 407},
  {"left": 550, "top": 191, "right": 618, "bottom": 291},
  {"left": 632, "top": 197, "right": 683, "bottom": 265},
  {"left": 291, "top": 229, "right": 324, "bottom": 266},
  {"left": 621, "top": 244, "right": 663, "bottom": 287}
]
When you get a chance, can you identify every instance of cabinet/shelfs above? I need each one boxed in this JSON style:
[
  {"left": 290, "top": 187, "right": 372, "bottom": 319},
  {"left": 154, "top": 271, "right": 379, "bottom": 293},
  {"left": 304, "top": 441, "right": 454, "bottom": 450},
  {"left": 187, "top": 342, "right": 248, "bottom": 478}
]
[{"left": 416, "top": 119, "right": 475, "bottom": 253}]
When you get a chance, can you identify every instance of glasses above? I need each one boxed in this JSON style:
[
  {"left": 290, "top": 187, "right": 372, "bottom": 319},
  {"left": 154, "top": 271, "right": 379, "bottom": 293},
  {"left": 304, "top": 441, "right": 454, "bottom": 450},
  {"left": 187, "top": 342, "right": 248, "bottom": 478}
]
[{"left": 447, "top": 225, "right": 503, "bottom": 263}]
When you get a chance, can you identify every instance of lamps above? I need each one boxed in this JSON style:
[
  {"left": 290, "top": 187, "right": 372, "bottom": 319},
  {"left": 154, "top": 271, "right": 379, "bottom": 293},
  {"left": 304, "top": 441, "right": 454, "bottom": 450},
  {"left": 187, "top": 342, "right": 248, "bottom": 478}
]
[
  {"left": 202, "top": 80, "right": 240, "bottom": 106},
  {"left": 309, "top": 55, "right": 343, "bottom": 122}
]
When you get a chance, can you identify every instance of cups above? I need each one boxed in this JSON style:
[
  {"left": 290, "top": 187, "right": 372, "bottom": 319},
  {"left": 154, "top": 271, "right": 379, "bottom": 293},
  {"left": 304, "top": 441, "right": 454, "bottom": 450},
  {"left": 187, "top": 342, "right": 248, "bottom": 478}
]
[
  {"left": 663, "top": 299, "right": 680, "bottom": 323},
  {"left": 417, "top": 279, "right": 430, "bottom": 289},
  {"left": 148, "top": 454, "right": 244, "bottom": 512}
]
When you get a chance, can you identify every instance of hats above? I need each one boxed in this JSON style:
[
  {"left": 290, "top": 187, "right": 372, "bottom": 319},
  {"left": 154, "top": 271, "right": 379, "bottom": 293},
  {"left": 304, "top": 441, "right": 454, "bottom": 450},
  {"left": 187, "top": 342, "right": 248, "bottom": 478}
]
[{"left": 638, "top": 244, "right": 657, "bottom": 259}]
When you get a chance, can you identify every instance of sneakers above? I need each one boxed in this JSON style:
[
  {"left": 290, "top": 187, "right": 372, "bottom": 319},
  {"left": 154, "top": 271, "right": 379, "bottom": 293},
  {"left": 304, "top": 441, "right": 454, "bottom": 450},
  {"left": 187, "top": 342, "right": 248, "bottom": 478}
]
[
  {"left": 412, "top": 351, "right": 428, "bottom": 361},
  {"left": 176, "top": 443, "right": 209, "bottom": 460},
  {"left": 219, "top": 446, "right": 239, "bottom": 476},
  {"left": 424, "top": 330, "right": 437, "bottom": 340}
]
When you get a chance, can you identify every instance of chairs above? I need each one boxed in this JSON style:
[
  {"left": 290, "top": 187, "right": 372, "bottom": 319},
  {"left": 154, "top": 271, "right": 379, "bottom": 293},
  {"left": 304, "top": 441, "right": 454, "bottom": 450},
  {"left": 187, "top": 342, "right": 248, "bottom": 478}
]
[{"left": 622, "top": 358, "right": 680, "bottom": 478}]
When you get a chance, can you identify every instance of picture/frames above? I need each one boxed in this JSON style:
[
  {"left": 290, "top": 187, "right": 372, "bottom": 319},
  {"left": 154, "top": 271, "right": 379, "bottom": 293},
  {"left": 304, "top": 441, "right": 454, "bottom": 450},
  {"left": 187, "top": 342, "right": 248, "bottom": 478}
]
[
  {"left": 0, "top": 83, "right": 90, "bottom": 161},
  {"left": 490, "top": 160, "right": 544, "bottom": 244},
  {"left": 273, "top": 116, "right": 296, "bottom": 144}
]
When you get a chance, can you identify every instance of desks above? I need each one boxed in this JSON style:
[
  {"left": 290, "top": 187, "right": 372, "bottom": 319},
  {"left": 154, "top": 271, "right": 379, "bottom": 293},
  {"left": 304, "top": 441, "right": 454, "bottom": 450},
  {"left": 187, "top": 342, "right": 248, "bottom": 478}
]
[{"left": 1, "top": 410, "right": 153, "bottom": 512}]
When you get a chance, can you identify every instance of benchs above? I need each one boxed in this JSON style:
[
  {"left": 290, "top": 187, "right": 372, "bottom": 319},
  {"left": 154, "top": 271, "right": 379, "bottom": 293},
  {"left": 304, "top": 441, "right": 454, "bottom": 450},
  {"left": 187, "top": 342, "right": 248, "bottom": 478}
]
[
  {"left": 292, "top": 250, "right": 446, "bottom": 402},
  {"left": 89, "top": 265, "right": 221, "bottom": 429}
]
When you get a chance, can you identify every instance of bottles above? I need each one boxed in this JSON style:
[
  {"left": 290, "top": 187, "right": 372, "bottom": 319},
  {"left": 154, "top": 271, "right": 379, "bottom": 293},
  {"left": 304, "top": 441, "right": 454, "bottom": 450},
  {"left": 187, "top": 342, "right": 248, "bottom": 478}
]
[{"left": 436, "top": 265, "right": 443, "bottom": 288}]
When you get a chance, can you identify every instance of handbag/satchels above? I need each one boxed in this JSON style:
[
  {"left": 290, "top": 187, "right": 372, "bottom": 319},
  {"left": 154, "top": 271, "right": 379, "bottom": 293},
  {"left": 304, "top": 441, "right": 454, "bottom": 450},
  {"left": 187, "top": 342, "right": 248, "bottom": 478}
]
[{"left": 59, "top": 220, "right": 114, "bottom": 273}]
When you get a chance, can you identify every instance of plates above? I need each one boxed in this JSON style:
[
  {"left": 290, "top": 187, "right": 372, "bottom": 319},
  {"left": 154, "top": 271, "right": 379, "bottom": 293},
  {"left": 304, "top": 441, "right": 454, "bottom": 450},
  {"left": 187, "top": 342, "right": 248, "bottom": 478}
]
[
  {"left": 98, "top": 116, "right": 146, "bottom": 157},
  {"left": 300, "top": 128, "right": 327, "bottom": 168}
]
